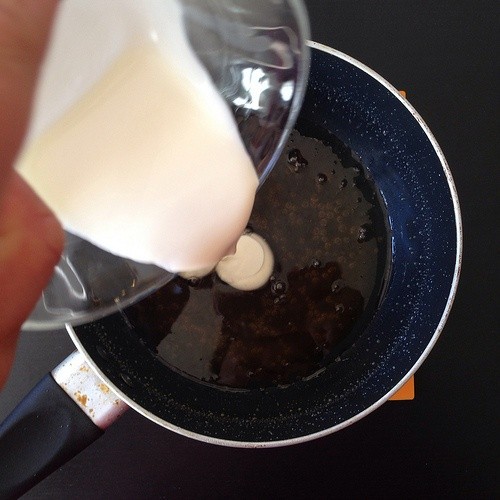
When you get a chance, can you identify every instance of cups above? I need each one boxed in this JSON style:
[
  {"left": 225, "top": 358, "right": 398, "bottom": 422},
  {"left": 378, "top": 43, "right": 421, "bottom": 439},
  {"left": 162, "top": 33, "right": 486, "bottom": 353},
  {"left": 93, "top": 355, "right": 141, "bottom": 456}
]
[{"left": 20, "top": 1, "right": 310, "bottom": 332}]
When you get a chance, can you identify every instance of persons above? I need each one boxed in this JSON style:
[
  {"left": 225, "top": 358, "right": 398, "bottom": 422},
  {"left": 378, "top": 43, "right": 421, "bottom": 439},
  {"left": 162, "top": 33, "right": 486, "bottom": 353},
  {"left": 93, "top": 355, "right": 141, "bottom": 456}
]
[{"left": 0, "top": 0, "right": 66, "bottom": 389}]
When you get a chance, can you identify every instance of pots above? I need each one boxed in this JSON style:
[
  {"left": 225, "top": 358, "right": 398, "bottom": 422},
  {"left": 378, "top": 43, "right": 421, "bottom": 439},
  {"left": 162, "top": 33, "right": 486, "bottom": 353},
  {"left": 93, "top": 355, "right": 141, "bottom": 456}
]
[{"left": 2, "top": 41, "right": 468, "bottom": 498}]
